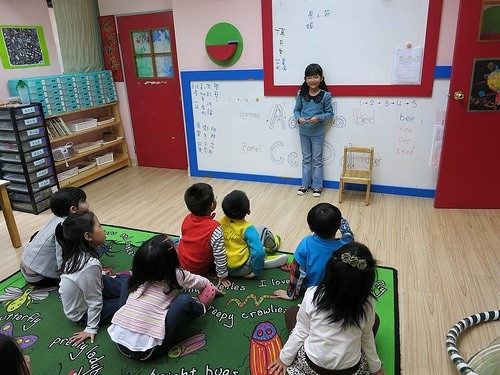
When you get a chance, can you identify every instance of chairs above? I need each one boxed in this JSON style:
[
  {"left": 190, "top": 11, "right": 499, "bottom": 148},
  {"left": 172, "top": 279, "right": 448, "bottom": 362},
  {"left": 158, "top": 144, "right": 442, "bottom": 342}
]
[{"left": 338, "top": 146, "right": 374, "bottom": 206}]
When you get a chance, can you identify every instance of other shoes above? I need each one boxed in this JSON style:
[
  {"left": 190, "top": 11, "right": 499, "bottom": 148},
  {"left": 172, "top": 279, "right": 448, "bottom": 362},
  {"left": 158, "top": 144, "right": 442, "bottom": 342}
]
[
  {"left": 197, "top": 283, "right": 216, "bottom": 307},
  {"left": 111, "top": 272, "right": 132, "bottom": 277}
]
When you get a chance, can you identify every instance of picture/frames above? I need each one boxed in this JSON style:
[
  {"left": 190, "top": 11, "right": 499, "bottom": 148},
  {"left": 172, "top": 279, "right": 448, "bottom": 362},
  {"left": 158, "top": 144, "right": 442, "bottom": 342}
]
[
  {"left": 466, "top": 58, "right": 500, "bottom": 112},
  {"left": 477, "top": 1, "right": 500, "bottom": 43}
]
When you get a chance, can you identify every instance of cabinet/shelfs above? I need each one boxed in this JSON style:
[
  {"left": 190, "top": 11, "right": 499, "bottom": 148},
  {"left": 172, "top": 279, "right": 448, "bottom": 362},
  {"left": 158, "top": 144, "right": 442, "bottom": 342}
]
[
  {"left": 45, "top": 102, "right": 132, "bottom": 188},
  {"left": 0, "top": 102, "right": 61, "bottom": 215}
]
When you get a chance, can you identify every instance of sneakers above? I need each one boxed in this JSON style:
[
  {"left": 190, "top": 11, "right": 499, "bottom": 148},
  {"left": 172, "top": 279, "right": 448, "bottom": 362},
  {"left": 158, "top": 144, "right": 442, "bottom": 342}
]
[
  {"left": 266, "top": 235, "right": 281, "bottom": 253},
  {"left": 297, "top": 186, "right": 311, "bottom": 195},
  {"left": 264, "top": 255, "right": 288, "bottom": 269},
  {"left": 312, "top": 191, "right": 321, "bottom": 196}
]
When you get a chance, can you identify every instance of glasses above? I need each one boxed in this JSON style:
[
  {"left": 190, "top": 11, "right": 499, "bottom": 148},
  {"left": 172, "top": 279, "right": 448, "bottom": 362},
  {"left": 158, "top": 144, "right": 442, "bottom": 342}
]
[{"left": 214, "top": 195, "right": 217, "bottom": 200}]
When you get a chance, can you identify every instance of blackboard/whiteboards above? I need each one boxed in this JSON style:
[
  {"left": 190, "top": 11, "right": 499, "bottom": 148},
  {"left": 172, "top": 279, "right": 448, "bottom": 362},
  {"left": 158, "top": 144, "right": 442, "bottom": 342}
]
[
  {"left": 270, "top": 0, "right": 431, "bottom": 87},
  {"left": 189, "top": 78, "right": 450, "bottom": 191}
]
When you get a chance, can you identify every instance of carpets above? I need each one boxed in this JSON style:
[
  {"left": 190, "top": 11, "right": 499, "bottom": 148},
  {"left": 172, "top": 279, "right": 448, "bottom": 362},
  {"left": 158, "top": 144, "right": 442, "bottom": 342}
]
[{"left": 0, "top": 222, "right": 400, "bottom": 375}]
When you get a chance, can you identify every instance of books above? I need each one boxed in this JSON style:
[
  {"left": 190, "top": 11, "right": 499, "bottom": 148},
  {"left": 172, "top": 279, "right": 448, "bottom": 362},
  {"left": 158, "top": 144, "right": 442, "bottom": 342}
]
[{"left": 46, "top": 118, "right": 73, "bottom": 140}]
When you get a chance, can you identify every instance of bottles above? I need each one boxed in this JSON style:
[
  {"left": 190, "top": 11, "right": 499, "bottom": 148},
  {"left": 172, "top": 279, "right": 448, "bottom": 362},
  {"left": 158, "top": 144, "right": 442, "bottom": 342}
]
[{"left": 15, "top": 80, "right": 30, "bottom": 104}]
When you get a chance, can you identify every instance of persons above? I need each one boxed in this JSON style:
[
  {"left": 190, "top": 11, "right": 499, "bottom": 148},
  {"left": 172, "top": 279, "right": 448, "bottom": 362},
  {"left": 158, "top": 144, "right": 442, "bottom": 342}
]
[
  {"left": 20, "top": 186, "right": 89, "bottom": 286},
  {"left": 275, "top": 202, "right": 354, "bottom": 300},
  {"left": 178, "top": 183, "right": 231, "bottom": 288},
  {"left": 107, "top": 234, "right": 225, "bottom": 361},
  {"left": 294, "top": 64, "right": 334, "bottom": 195},
  {"left": 267, "top": 242, "right": 384, "bottom": 375},
  {"left": 0, "top": 334, "right": 31, "bottom": 375},
  {"left": 217, "top": 190, "right": 288, "bottom": 279},
  {"left": 56, "top": 211, "right": 121, "bottom": 342}
]
